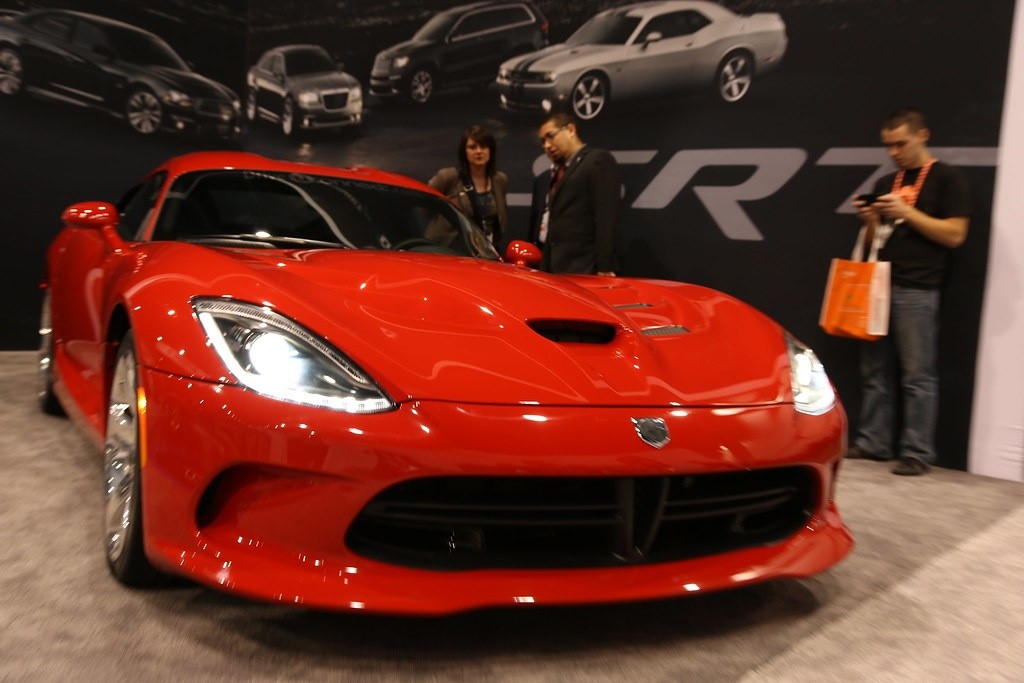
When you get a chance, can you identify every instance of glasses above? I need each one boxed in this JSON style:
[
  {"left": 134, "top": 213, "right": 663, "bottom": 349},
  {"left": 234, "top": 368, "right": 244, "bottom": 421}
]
[{"left": 539, "top": 129, "right": 561, "bottom": 145}]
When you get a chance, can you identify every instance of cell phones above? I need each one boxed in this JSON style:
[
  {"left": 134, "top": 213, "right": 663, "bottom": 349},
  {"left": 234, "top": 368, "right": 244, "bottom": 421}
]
[{"left": 858, "top": 193, "right": 886, "bottom": 224}]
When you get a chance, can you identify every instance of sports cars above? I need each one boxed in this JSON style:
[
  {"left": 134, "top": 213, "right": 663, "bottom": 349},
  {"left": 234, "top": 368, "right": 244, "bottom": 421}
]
[{"left": 31, "top": 152, "right": 855, "bottom": 620}]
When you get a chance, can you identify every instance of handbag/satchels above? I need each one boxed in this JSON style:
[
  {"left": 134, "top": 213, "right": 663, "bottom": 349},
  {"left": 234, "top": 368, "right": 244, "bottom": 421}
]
[{"left": 818, "top": 216, "right": 892, "bottom": 342}]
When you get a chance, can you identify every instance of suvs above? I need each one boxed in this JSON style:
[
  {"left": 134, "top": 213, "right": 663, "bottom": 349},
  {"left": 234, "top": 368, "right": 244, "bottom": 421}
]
[{"left": 367, "top": 0, "right": 550, "bottom": 115}]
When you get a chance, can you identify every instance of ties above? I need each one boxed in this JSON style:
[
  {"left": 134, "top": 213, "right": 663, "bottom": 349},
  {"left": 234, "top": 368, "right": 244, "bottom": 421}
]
[{"left": 549, "top": 166, "right": 565, "bottom": 202}]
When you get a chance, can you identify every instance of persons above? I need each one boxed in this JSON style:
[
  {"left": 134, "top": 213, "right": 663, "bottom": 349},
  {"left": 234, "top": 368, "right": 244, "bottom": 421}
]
[
  {"left": 417, "top": 125, "right": 510, "bottom": 260},
  {"left": 845, "top": 110, "right": 974, "bottom": 477},
  {"left": 534, "top": 112, "right": 621, "bottom": 277}
]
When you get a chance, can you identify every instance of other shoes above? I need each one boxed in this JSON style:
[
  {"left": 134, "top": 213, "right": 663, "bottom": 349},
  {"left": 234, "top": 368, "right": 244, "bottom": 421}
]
[
  {"left": 842, "top": 442, "right": 890, "bottom": 461},
  {"left": 891, "top": 457, "right": 933, "bottom": 476}
]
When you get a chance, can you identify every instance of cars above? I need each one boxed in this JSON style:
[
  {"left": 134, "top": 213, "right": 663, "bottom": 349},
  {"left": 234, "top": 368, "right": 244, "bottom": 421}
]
[
  {"left": 247, "top": 44, "right": 364, "bottom": 139},
  {"left": 494, "top": 0, "right": 789, "bottom": 133},
  {"left": 0, "top": 3, "right": 247, "bottom": 148}
]
